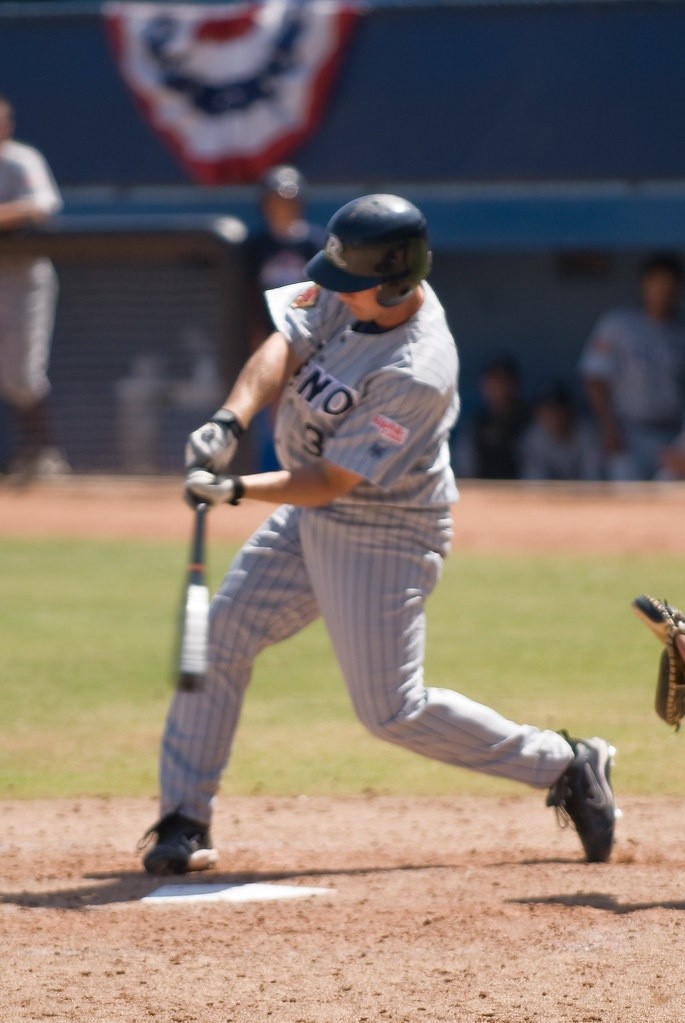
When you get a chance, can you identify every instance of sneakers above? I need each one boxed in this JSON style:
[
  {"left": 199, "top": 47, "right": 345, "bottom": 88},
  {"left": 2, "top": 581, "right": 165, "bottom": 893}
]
[
  {"left": 135, "top": 810, "right": 218, "bottom": 873},
  {"left": 545, "top": 728, "right": 618, "bottom": 862}
]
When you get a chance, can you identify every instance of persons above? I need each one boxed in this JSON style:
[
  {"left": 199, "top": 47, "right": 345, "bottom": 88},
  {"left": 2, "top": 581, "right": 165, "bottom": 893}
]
[
  {"left": 580, "top": 261, "right": 685, "bottom": 481},
  {"left": 218, "top": 166, "right": 321, "bottom": 474},
  {"left": 142, "top": 194, "right": 615, "bottom": 873},
  {"left": 467, "top": 352, "right": 530, "bottom": 480},
  {"left": 0, "top": 99, "right": 62, "bottom": 489},
  {"left": 519, "top": 387, "right": 602, "bottom": 482}
]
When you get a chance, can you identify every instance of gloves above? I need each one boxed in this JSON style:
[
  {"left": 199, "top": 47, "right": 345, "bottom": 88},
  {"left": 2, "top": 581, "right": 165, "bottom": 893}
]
[
  {"left": 183, "top": 470, "right": 245, "bottom": 507},
  {"left": 185, "top": 409, "right": 243, "bottom": 473}
]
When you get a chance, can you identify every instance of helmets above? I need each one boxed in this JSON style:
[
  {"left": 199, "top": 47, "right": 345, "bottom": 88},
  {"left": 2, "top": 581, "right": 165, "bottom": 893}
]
[{"left": 303, "top": 192, "right": 430, "bottom": 307}]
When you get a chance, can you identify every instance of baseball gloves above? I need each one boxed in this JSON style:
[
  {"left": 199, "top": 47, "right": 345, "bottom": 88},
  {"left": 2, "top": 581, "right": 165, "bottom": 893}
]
[{"left": 631, "top": 593, "right": 685, "bottom": 726}]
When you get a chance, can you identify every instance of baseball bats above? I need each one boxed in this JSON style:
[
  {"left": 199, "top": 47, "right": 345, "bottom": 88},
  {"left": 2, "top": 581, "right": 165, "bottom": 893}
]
[{"left": 168, "top": 467, "right": 214, "bottom": 692}]
